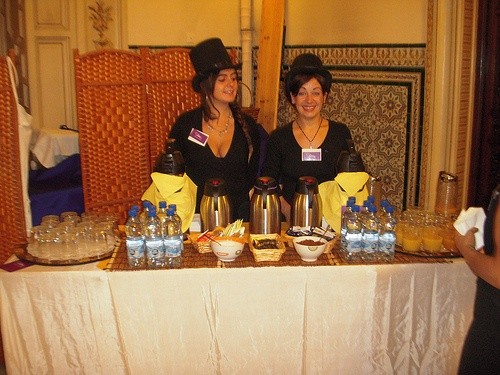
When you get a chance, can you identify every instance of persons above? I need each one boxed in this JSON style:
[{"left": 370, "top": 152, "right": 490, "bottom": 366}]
[
  {"left": 454, "top": 101, "right": 500, "bottom": 375},
  {"left": 158, "top": 38, "right": 261, "bottom": 222},
  {"left": 259, "top": 53, "right": 373, "bottom": 227}
]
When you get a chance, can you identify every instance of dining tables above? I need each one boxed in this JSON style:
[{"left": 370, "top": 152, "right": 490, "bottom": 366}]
[{"left": 0, "top": 235, "right": 485, "bottom": 375}]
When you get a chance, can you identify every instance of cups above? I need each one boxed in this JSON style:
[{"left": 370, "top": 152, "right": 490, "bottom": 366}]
[
  {"left": 31, "top": 212, "right": 119, "bottom": 260},
  {"left": 394, "top": 209, "right": 459, "bottom": 252}
]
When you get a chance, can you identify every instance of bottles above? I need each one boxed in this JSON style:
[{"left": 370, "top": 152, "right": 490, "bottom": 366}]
[
  {"left": 340, "top": 196, "right": 397, "bottom": 262},
  {"left": 124, "top": 201, "right": 184, "bottom": 270}
]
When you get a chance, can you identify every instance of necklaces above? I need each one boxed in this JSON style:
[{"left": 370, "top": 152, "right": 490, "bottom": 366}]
[
  {"left": 203, "top": 111, "right": 232, "bottom": 135},
  {"left": 295, "top": 117, "right": 322, "bottom": 143}
]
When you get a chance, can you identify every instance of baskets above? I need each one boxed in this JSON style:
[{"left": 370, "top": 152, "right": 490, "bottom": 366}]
[
  {"left": 282, "top": 233, "right": 338, "bottom": 253},
  {"left": 189, "top": 231, "right": 213, "bottom": 254},
  {"left": 248, "top": 233, "right": 287, "bottom": 262}
]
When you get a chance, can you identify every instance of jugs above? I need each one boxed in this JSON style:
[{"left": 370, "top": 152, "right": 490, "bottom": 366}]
[
  {"left": 289, "top": 175, "right": 323, "bottom": 228},
  {"left": 248, "top": 177, "right": 282, "bottom": 236},
  {"left": 199, "top": 177, "right": 231, "bottom": 233}
]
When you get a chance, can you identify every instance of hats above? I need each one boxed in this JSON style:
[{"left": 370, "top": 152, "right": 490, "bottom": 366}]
[
  {"left": 284, "top": 52, "right": 333, "bottom": 87},
  {"left": 188, "top": 37, "right": 243, "bottom": 93}
]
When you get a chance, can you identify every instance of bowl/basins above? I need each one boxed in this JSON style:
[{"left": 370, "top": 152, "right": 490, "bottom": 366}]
[
  {"left": 210, "top": 239, "right": 244, "bottom": 263},
  {"left": 292, "top": 236, "right": 328, "bottom": 263}
]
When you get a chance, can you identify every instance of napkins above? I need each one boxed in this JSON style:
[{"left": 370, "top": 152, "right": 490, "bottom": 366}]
[
  {"left": 142, "top": 172, "right": 198, "bottom": 233},
  {"left": 315, "top": 172, "right": 370, "bottom": 234}
]
